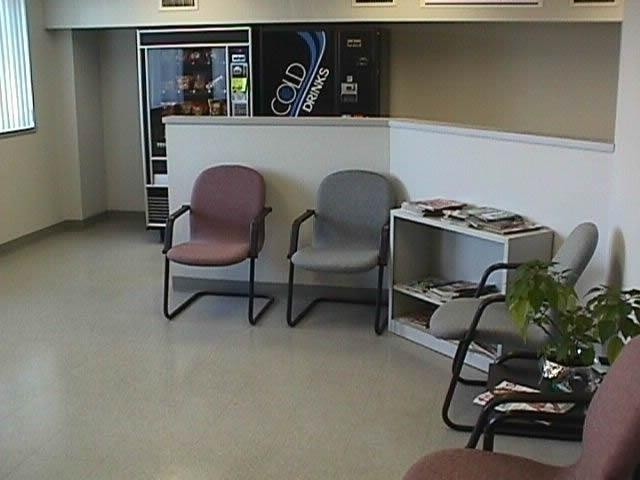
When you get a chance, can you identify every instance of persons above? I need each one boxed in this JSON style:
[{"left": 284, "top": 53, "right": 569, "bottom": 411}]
[{"left": 489, "top": 386, "right": 564, "bottom": 411}]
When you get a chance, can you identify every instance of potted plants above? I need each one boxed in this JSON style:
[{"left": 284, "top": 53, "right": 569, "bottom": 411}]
[{"left": 505, "top": 259, "right": 640, "bottom": 393}]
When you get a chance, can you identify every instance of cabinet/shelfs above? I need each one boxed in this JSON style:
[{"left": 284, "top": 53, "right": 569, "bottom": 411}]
[{"left": 388, "top": 208, "right": 553, "bottom": 374}]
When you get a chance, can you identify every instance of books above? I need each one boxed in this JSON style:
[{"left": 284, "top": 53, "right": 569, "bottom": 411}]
[
  {"left": 397, "top": 311, "right": 497, "bottom": 359},
  {"left": 473, "top": 379, "right": 574, "bottom": 427},
  {"left": 396, "top": 276, "right": 499, "bottom": 303},
  {"left": 400, "top": 198, "right": 543, "bottom": 235}
]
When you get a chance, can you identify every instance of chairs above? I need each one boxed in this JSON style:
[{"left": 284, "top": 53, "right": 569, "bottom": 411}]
[
  {"left": 286, "top": 169, "right": 397, "bottom": 335},
  {"left": 401, "top": 335, "right": 640, "bottom": 480},
  {"left": 161, "top": 164, "right": 274, "bottom": 325},
  {"left": 429, "top": 222, "right": 599, "bottom": 432}
]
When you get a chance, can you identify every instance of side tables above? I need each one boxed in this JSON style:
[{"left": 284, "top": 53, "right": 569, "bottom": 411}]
[{"left": 481, "top": 351, "right": 610, "bottom": 453}]
